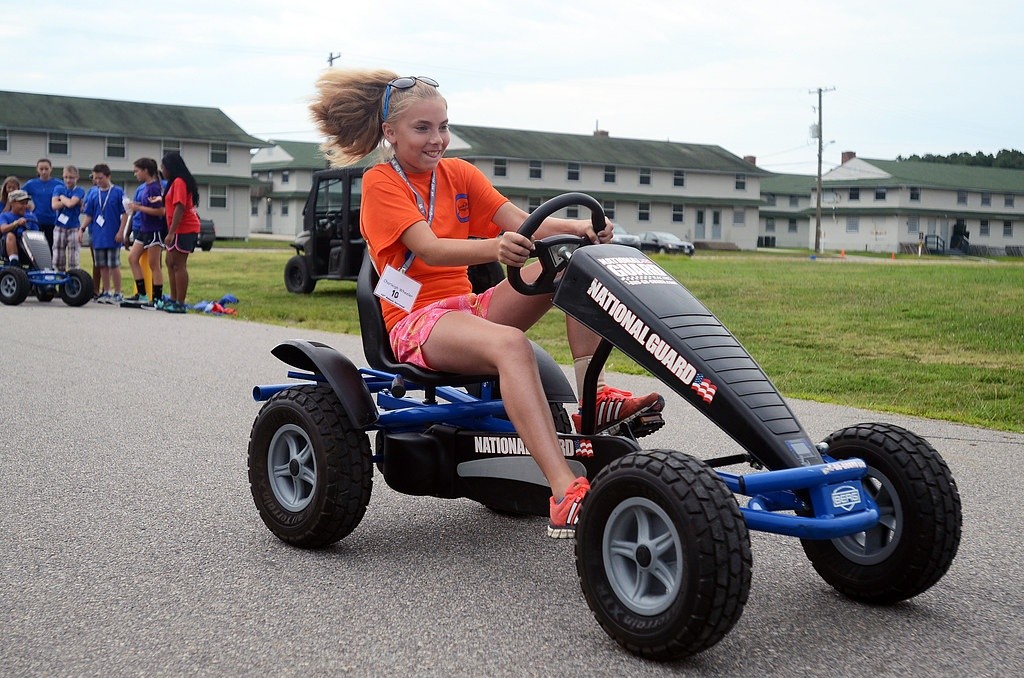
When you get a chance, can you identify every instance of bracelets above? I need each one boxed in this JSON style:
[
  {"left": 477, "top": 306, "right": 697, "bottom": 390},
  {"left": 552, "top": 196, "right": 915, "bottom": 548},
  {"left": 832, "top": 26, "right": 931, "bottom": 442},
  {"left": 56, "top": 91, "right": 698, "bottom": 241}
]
[{"left": 80, "top": 227, "right": 85, "bottom": 232}]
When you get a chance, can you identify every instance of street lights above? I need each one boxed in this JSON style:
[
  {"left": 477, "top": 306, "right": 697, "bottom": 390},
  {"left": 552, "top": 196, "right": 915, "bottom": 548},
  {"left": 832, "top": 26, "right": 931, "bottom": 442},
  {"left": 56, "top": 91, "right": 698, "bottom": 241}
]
[{"left": 815, "top": 139, "right": 836, "bottom": 251}]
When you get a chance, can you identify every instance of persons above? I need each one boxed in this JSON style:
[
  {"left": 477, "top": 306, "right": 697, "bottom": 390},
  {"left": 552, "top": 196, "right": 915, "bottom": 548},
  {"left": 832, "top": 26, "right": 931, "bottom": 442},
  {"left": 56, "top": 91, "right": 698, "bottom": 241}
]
[
  {"left": 21, "top": 159, "right": 64, "bottom": 270},
  {"left": 0, "top": 188, "right": 39, "bottom": 267},
  {"left": 311, "top": 68, "right": 665, "bottom": 540},
  {"left": 0, "top": 175, "right": 35, "bottom": 214},
  {"left": 76, "top": 164, "right": 128, "bottom": 304},
  {"left": 121, "top": 157, "right": 168, "bottom": 310},
  {"left": 147, "top": 152, "right": 201, "bottom": 313},
  {"left": 52, "top": 165, "right": 85, "bottom": 274}
]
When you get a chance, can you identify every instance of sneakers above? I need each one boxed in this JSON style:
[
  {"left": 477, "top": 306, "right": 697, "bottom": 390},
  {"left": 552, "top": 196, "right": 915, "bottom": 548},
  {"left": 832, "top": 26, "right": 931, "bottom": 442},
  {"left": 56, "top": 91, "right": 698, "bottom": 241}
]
[
  {"left": 97, "top": 293, "right": 112, "bottom": 303},
  {"left": 154, "top": 299, "right": 164, "bottom": 310},
  {"left": 122, "top": 292, "right": 149, "bottom": 306},
  {"left": 164, "top": 301, "right": 186, "bottom": 312},
  {"left": 547, "top": 477, "right": 591, "bottom": 539},
  {"left": 106, "top": 293, "right": 124, "bottom": 304},
  {"left": 572, "top": 385, "right": 665, "bottom": 436}
]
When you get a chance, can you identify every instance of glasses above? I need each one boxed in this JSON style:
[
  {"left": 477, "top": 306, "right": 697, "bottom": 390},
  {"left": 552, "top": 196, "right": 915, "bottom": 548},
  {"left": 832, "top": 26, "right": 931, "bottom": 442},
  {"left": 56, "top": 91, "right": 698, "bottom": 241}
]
[
  {"left": 15, "top": 200, "right": 28, "bottom": 205},
  {"left": 383, "top": 77, "right": 439, "bottom": 121}
]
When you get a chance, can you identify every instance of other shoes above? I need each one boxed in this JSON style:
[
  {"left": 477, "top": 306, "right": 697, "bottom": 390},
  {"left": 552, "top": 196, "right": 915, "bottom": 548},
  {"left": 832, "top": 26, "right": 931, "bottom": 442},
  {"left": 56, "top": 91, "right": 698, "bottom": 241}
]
[{"left": 10, "top": 259, "right": 24, "bottom": 270}]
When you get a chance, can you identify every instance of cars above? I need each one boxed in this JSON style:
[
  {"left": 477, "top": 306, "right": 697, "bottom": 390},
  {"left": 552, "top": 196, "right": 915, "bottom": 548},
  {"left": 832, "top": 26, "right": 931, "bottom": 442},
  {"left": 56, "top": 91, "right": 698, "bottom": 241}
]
[
  {"left": 610, "top": 223, "right": 642, "bottom": 251},
  {"left": 636, "top": 231, "right": 696, "bottom": 258}
]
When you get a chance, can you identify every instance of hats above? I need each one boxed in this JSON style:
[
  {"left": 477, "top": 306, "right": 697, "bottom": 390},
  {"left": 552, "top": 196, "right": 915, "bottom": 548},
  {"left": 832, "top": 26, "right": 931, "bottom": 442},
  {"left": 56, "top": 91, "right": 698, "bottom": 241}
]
[
  {"left": 89, "top": 174, "right": 93, "bottom": 179},
  {"left": 8, "top": 190, "right": 31, "bottom": 202}
]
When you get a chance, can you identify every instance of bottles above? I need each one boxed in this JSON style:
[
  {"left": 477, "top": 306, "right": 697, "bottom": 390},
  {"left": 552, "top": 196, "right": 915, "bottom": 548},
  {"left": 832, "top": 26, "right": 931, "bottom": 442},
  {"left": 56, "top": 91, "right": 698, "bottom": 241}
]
[
  {"left": 205, "top": 300, "right": 214, "bottom": 312},
  {"left": 122, "top": 196, "right": 133, "bottom": 214}
]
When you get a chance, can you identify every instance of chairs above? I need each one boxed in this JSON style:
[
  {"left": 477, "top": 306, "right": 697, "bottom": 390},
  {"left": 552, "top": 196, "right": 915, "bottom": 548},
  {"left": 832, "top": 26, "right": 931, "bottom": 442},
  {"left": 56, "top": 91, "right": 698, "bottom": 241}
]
[{"left": 356, "top": 243, "right": 497, "bottom": 386}]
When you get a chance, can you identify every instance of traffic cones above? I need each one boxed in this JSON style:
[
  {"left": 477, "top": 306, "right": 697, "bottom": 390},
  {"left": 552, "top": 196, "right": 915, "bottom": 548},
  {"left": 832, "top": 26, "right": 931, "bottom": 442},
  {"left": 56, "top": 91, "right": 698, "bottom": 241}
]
[
  {"left": 892, "top": 251, "right": 896, "bottom": 260},
  {"left": 841, "top": 249, "right": 846, "bottom": 257}
]
